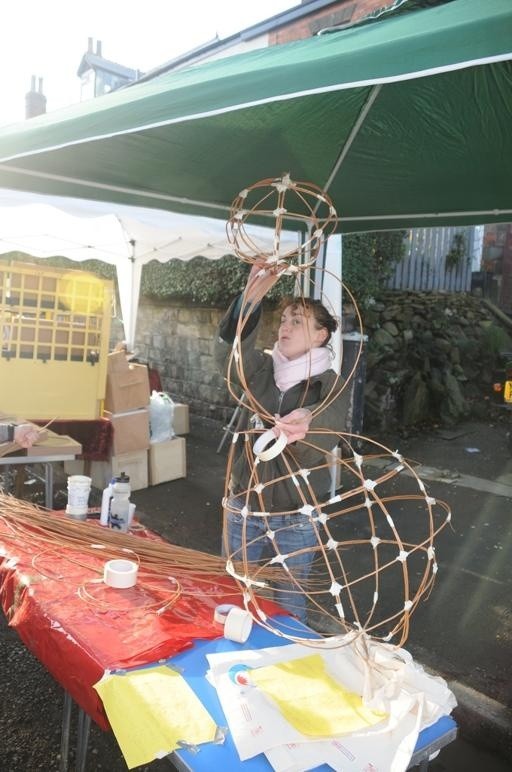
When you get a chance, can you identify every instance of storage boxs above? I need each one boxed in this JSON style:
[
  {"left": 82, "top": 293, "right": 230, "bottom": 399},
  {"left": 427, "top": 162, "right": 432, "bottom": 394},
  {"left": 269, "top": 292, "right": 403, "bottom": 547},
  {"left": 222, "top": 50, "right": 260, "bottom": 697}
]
[{"left": 62, "top": 362, "right": 190, "bottom": 493}]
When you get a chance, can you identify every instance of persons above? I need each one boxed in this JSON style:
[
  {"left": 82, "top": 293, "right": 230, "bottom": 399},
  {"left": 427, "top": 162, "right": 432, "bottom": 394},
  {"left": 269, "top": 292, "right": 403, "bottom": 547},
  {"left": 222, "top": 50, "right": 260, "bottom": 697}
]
[
  {"left": 216, "top": 256, "right": 349, "bottom": 624},
  {"left": 0, "top": 423, "right": 42, "bottom": 448}
]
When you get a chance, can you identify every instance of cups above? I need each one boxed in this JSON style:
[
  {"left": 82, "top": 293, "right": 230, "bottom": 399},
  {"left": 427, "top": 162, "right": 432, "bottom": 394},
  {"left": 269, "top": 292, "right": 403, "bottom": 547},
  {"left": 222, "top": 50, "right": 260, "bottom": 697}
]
[{"left": 65, "top": 475, "right": 91, "bottom": 521}]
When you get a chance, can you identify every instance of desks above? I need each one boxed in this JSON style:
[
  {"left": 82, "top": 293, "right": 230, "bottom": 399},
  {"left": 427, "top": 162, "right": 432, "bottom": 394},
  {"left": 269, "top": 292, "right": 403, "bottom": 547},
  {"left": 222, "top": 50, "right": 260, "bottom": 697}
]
[
  {"left": 0, "top": 454, "right": 76, "bottom": 509},
  {"left": 0, "top": 506, "right": 460, "bottom": 771}
]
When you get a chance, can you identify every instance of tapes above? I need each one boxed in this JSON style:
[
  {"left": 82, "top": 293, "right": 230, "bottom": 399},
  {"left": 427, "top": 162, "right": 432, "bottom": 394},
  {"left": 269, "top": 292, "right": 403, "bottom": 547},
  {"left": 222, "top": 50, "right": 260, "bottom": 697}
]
[
  {"left": 213, "top": 604, "right": 238, "bottom": 624},
  {"left": 103, "top": 559, "right": 138, "bottom": 588},
  {"left": 224, "top": 608, "right": 253, "bottom": 643}
]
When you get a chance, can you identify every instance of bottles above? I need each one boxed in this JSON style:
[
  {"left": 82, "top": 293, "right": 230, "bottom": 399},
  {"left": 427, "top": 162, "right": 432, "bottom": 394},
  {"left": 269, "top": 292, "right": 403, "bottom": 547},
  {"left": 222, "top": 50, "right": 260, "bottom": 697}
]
[{"left": 100, "top": 472, "right": 130, "bottom": 533}]
